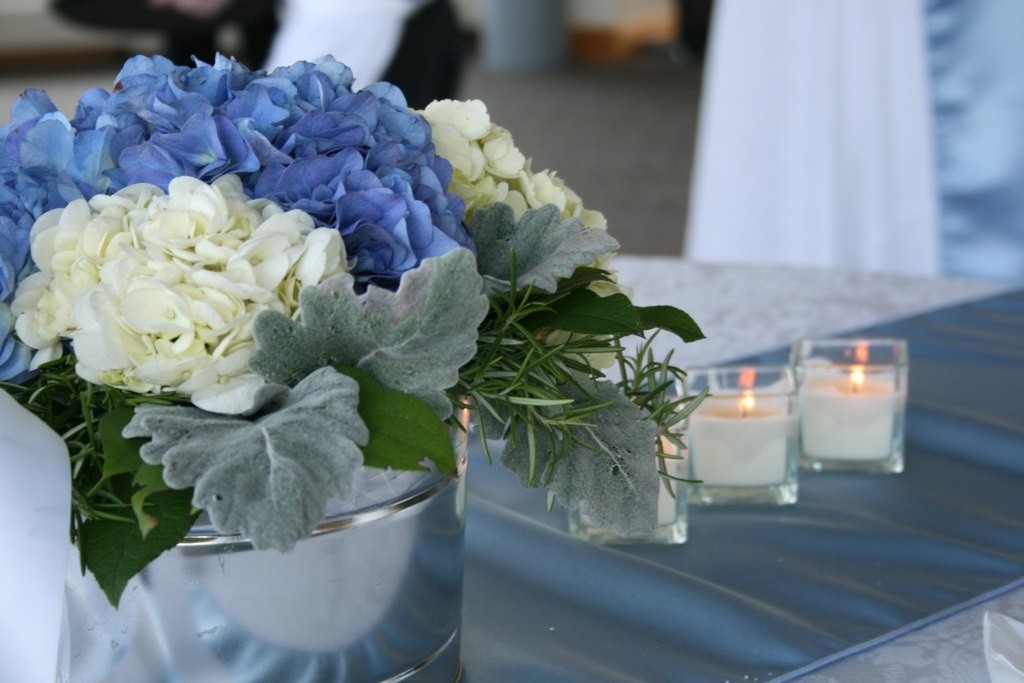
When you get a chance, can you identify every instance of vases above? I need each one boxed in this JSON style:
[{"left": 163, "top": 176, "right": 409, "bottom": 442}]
[{"left": 136, "top": 396, "right": 469, "bottom": 683}]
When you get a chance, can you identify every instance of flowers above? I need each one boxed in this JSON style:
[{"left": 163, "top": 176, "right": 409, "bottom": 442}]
[{"left": 0, "top": 54, "right": 712, "bottom": 610}]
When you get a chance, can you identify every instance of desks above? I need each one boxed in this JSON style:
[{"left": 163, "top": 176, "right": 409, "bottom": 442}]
[{"left": 0, "top": 253, "right": 1024, "bottom": 682}]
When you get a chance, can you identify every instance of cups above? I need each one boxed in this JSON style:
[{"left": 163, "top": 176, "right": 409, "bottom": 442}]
[
  {"left": 674, "top": 363, "right": 801, "bottom": 506},
  {"left": 789, "top": 336, "right": 910, "bottom": 473},
  {"left": 568, "top": 393, "right": 692, "bottom": 546}
]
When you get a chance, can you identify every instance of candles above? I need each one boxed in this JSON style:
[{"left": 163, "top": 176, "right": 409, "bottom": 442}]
[
  {"left": 798, "top": 370, "right": 895, "bottom": 459},
  {"left": 688, "top": 390, "right": 789, "bottom": 486}
]
